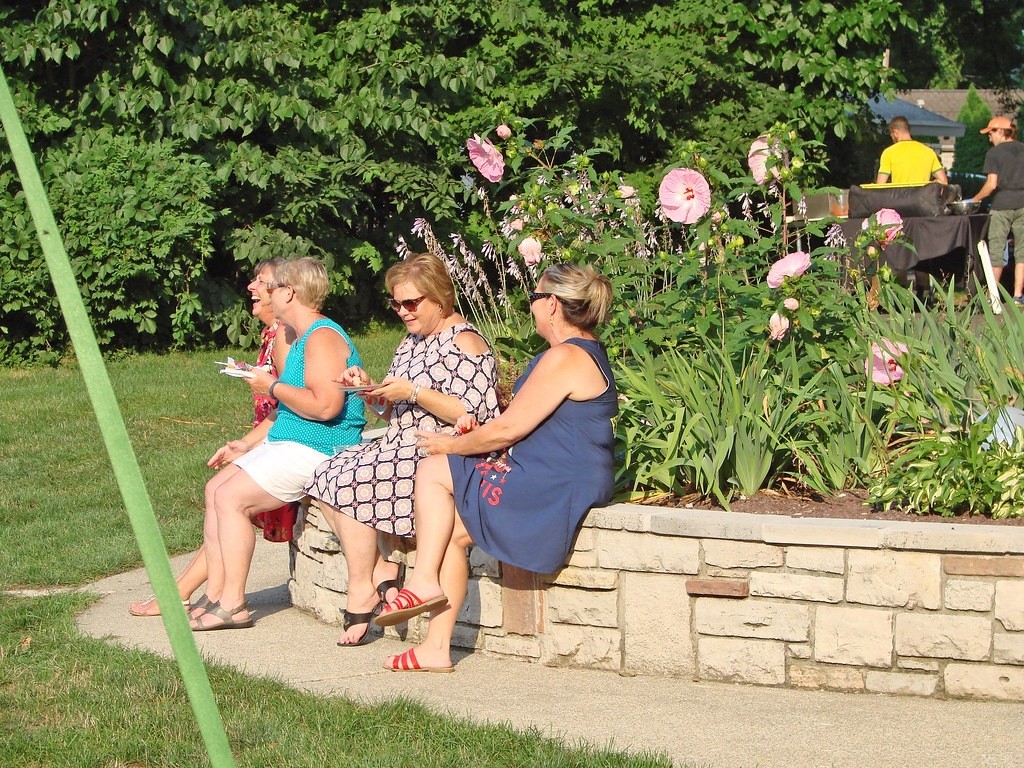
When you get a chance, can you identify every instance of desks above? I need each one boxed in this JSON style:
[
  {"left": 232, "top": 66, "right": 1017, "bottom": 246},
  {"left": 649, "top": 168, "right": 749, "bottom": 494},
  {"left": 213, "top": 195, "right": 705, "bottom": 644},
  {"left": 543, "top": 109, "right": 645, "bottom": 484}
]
[{"left": 786, "top": 213, "right": 1003, "bottom": 315}]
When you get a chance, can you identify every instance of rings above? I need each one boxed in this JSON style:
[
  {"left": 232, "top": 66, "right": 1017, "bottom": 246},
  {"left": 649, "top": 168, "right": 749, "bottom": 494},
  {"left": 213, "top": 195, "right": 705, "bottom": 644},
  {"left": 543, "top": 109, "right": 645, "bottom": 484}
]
[{"left": 417, "top": 448, "right": 430, "bottom": 458}]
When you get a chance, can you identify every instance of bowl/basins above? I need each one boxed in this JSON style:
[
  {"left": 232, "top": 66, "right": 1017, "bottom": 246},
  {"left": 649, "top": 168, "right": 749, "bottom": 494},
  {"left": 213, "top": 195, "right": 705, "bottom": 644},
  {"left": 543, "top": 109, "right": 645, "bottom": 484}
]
[{"left": 946, "top": 201, "right": 980, "bottom": 215}]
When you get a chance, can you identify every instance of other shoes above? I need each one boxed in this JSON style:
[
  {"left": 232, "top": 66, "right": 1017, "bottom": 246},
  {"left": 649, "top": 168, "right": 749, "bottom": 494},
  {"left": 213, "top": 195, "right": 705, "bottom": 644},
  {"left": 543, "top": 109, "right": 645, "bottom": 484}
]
[
  {"left": 877, "top": 295, "right": 913, "bottom": 314},
  {"left": 960, "top": 299, "right": 995, "bottom": 315},
  {"left": 913, "top": 296, "right": 931, "bottom": 313},
  {"left": 1013, "top": 295, "right": 1024, "bottom": 307}
]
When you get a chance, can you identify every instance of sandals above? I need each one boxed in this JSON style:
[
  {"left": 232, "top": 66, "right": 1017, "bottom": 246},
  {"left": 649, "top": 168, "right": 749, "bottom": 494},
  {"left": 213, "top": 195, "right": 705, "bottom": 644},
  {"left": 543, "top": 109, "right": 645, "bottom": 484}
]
[
  {"left": 188, "top": 594, "right": 221, "bottom": 620},
  {"left": 129, "top": 594, "right": 155, "bottom": 611},
  {"left": 132, "top": 598, "right": 190, "bottom": 616},
  {"left": 190, "top": 599, "right": 253, "bottom": 631}
]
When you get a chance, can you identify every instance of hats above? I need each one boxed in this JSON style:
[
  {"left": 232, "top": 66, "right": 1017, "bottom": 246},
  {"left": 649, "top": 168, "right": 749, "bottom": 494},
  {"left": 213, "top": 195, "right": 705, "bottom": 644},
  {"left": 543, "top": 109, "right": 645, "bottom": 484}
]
[{"left": 980, "top": 117, "right": 1018, "bottom": 134}]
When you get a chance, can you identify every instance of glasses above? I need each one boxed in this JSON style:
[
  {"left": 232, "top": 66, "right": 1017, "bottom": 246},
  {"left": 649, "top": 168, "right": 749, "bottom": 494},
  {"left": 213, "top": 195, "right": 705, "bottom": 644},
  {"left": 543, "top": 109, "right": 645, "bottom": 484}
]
[
  {"left": 267, "top": 283, "right": 295, "bottom": 294},
  {"left": 386, "top": 295, "right": 428, "bottom": 312},
  {"left": 988, "top": 132, "right": 993, "bottom": 138},
  {"left": 529, "top": 291, "right": 569, "bottom": 306}
]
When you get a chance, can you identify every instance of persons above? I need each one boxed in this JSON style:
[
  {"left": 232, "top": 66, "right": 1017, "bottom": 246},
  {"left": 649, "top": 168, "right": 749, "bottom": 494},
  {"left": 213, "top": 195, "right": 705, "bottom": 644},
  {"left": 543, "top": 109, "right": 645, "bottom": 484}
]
[
  {"left": 373, "top": 260, "right": 621, "bottom": 672},
  {"left": 970, "top": 116, "right": 1024, "bottom": 309},
  {"left": 181, "top": 254, "right": 365, "bottom": 632},
  {"left": 303, "top": 253, "right": 504, "bottom": 647},
  {"left": 127, "top": 258, "right": 303, "bottom": 618},
  {"left": 875, "top": 115, "right": 949, "bottom": 185}
]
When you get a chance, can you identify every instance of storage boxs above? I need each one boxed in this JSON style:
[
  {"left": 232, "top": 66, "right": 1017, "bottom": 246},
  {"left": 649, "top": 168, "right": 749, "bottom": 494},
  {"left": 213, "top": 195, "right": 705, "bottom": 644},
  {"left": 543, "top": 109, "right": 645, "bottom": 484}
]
[{"left": 793, "top": 189, "right": 849, "bottom": 216}]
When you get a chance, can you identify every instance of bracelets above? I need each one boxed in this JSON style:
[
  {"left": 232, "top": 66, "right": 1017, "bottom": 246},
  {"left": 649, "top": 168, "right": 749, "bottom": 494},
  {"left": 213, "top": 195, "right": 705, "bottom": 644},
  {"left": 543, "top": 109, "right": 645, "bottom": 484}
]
[
  {"left": 411, "top": 381, "right": 421, "bottom": 402},
  {"left": 269, "top": 380, "right": 282, "bottom": 399}
]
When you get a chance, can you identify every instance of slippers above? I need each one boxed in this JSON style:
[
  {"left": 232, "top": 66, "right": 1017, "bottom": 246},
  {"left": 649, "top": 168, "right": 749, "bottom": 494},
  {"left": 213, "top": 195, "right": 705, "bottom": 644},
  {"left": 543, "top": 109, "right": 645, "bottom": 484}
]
[
  {"left": 376, "top": 562, "right": 406, "bottom": 605},
  {"left": 374, "top": 588, "right": 448, "bottom": 626},
  {"left": 383, "top": 648, "right": 455, "bottom": 673},
  {"left": 337, "top": 600, "right": 382, "bottom": 646}
]
